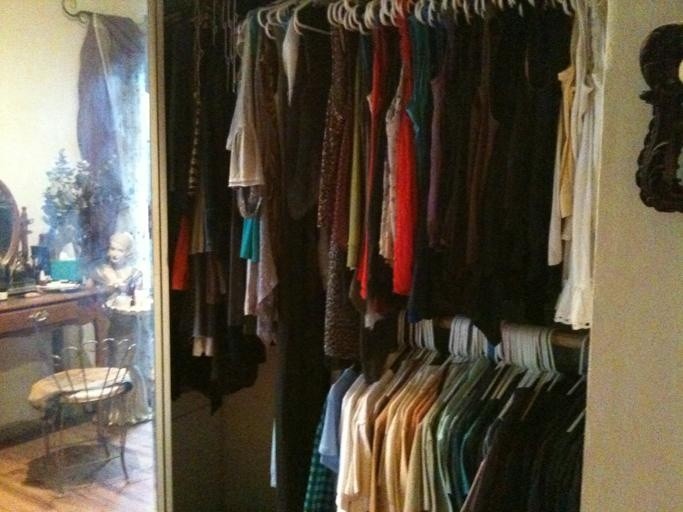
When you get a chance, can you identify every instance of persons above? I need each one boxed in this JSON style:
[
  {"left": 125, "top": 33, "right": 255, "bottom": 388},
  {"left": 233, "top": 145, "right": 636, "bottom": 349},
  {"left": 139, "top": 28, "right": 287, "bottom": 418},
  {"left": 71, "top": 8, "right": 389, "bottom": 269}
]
[{"left": 85, "top": 232, "right": 151, "bottom": 427}]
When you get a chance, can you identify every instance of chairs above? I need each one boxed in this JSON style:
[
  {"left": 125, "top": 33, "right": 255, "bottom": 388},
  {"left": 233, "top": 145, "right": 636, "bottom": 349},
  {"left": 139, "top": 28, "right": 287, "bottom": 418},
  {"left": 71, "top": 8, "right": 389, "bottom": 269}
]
[{"left": 27, "top": 310, "right": 140, "bottom": 497}]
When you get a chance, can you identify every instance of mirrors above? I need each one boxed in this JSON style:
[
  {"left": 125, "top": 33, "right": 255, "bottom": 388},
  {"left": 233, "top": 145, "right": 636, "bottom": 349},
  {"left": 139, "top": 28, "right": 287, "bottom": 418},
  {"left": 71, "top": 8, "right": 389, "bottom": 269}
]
[{"left": 0, "top": 179, "right": 31, "bottom": 286}]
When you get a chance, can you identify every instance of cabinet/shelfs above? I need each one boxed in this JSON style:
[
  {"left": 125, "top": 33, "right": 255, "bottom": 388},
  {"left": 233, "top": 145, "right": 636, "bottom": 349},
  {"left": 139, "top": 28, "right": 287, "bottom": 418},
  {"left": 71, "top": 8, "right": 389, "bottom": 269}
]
[{"left": 146, "top": 0, "right": 682, "bottom": 511}]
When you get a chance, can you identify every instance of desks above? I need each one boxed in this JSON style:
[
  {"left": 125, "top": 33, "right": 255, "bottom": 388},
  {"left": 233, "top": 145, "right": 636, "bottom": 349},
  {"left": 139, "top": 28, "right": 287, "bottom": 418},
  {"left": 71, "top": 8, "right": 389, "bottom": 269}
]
[{"left": 0, "top": 281, "right": 117, "bottom": 437}]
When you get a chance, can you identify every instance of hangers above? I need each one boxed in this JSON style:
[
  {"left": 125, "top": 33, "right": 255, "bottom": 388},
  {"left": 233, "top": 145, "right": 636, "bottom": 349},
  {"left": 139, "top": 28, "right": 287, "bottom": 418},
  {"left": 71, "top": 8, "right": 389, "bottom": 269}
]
[{"left": 396, "top": 307, "right": 590, "bottom": 434}]
